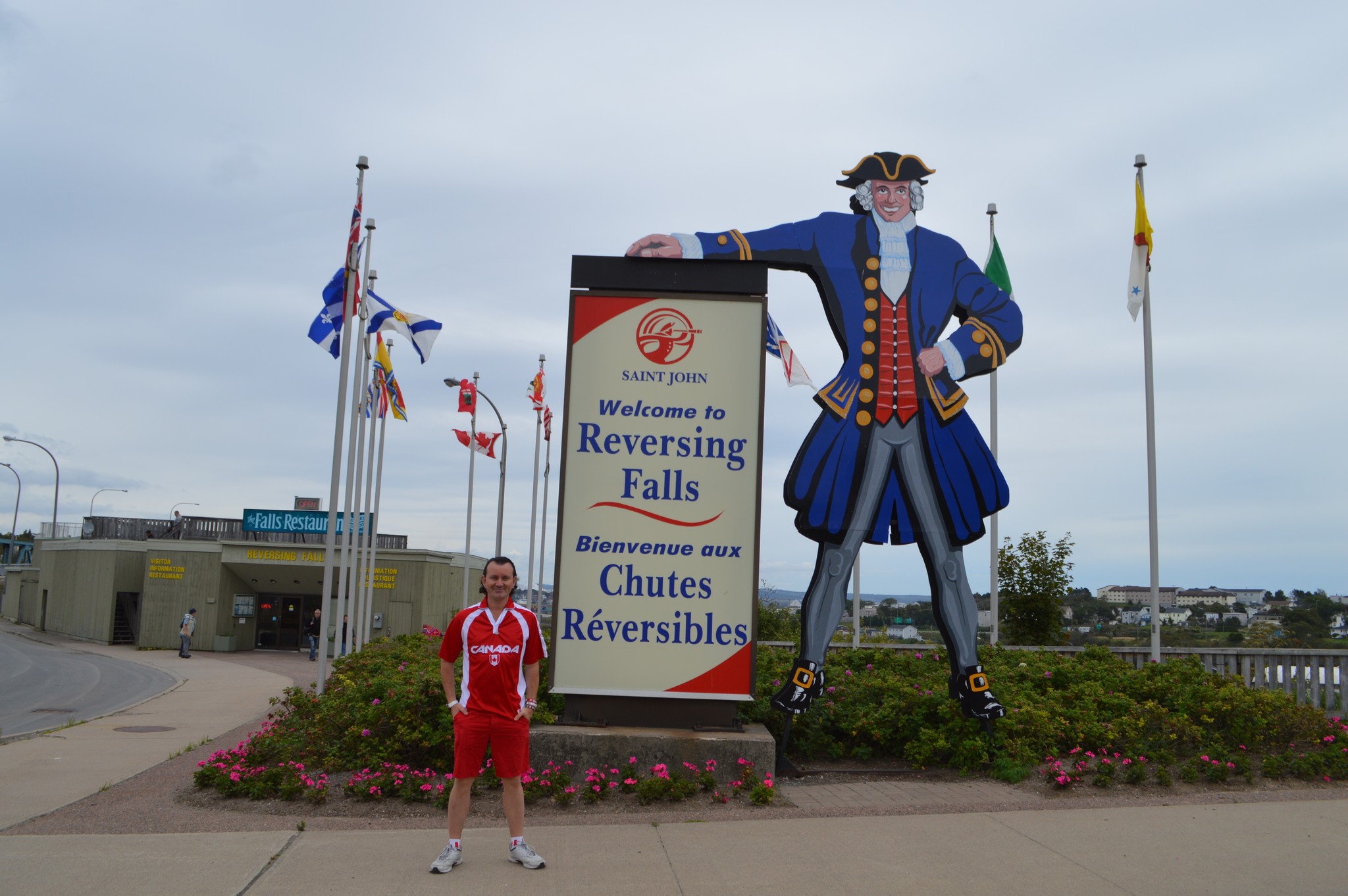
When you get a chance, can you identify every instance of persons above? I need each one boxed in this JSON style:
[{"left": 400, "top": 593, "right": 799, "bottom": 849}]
[
  {"left": 333, "top": 615, "right": 356, "bottom": 657},
  {"left": 308, "top": 609, "right": 321, "bottom": 662},
  {"left": 171, "top": 511, "right": 182, "bottom": 539},
  {"left": 428, "top": 557, "right": 548, "bottom": 873},
  {"left": 178, "top": 608, "right": 197, "bottom": 658}
]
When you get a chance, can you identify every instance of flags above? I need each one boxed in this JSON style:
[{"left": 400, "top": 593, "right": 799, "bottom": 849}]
[
  {"left": 765, "top": 314, "right": 815, "bottom": 395},
  {"left": 982, "top": 217, "right": 1014, "bottom": 307},
  {"left": 306, "top": 179, "right": 553, "bottom": 459},
  {"left": 1127, "top": 172, "right": 1154, "bottom": 322}
]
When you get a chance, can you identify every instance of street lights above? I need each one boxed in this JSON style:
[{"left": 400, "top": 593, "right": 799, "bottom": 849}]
[
  {"left": 169, "top": 503, "right": 199, "bottom": 520},
  {"left": 0, "top": 435, "right": 59, "bottom": 539},
  {"left": 90, "top": 489, "right": 128, "bottom": 516},
  {"left": 444, "top": 377, "right": 507, "bottom": 559}
]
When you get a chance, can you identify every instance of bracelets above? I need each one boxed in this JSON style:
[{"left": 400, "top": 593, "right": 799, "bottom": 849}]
[
  {"left": 446, "top": 700, "right": 458, "bottom": 710},
  {"left": 525, "top": 699, "right": 537, "bottom": 711}
]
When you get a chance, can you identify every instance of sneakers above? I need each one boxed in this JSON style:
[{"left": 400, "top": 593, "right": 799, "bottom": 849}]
[
  {"left": 427, "top": 843, "right": 463, "bottom": 872},
  {"left": 508, "top": 842, "right": 546, "bottom": 869}
]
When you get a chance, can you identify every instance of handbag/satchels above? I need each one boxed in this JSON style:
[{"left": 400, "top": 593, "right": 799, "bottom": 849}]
[
  {"left": 179, "top": 615, "right": 190, "bottom": 629},
  {"left": 302, "top": 626, "right": 312, "bottom": 636}
]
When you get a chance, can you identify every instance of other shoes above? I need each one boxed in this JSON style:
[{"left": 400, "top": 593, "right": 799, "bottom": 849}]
[
  {"left": 181, "top": 655, "right": 185, "bottom": 658},
  {"left": 179, "top": 654, "right": 181, "bottom": 656},
  {"left": 185, "top": 654, "right": 191, "bottom": 658},
  {"left": 309, "top": 657, "right": 315, "bottom": 661}
]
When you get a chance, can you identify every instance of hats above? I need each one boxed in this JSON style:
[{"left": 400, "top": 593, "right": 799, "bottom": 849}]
[{"left": 189, "top": 608, "right": 196, "bottom": 614}]
